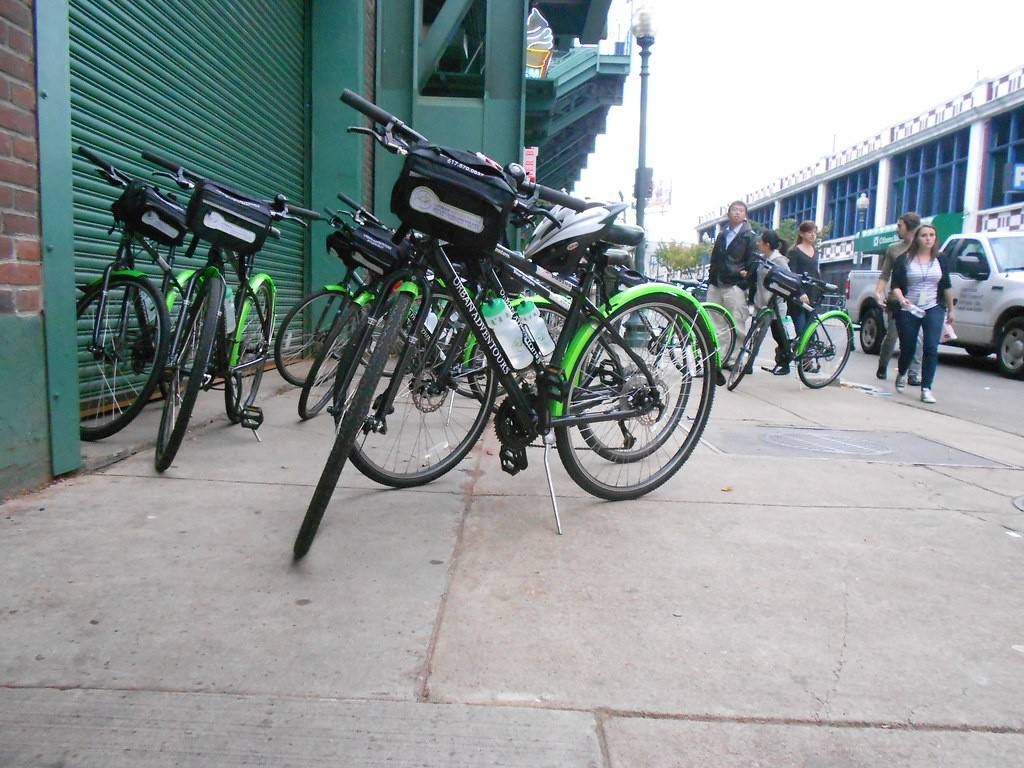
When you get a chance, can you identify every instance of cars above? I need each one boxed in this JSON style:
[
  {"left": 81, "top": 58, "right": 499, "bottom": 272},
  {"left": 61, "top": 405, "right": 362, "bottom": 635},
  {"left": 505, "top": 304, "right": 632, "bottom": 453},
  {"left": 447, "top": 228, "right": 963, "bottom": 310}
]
[{"left": 691, "top": 280, "right": 711, "bottom": 302}]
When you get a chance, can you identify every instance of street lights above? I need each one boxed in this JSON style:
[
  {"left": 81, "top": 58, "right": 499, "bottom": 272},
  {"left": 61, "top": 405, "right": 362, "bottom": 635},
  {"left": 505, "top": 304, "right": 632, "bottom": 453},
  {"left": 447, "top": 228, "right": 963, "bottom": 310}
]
[
  {"left": 856, "top": 192, "right": 869, "bottom": 267},
  {"left": 700, "top": 231, "right": 709, "bottom": 281},
  {"left": 624, "top": 8, "right": 660, "bottom": 348}
]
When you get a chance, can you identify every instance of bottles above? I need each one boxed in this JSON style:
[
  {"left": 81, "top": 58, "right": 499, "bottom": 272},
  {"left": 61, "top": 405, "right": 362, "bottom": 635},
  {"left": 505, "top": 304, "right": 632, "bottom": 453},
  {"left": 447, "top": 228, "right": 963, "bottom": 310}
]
[
  {"left": 224, "top": 288, "right": 236, "bottom": 334},
  {"left": 413, "top": 305, "right": 447, "bottom": 340},
  {"left": 517, "top": 300, "right": 555, "bottom": 356},
  {"left": 140, "top": 291, "right": 156, "bottom": 323},
  {"left": 907, "top": 303, "right": 925, "bottom": 318},
  {"left": 482, "top": 298, "right": 534, "bottom": 369},
  {"left": 783, "top": 316, "right": 796, "bottom": 339},
  {"left": 438, "top": 299, "right": 466, "bottom": 328}
]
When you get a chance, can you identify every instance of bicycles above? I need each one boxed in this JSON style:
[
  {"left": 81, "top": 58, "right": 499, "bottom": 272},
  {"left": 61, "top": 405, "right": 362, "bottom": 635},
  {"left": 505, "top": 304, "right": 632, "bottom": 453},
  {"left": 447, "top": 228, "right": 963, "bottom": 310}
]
[
  {"left": 273, "top": 87, "right": 728, "bottom": 560},
  {"left": 74, "top": 144, "right": 322, "bottom": 475},
  {"left": 607, "top": 256, "right": 857, "bottom": 391}
]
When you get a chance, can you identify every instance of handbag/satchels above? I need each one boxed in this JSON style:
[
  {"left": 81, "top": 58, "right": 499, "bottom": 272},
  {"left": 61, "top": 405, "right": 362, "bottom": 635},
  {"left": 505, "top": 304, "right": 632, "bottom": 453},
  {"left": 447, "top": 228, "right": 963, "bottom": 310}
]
[{"left": 939, "top": 313, "right": 957, "bottom": 344}]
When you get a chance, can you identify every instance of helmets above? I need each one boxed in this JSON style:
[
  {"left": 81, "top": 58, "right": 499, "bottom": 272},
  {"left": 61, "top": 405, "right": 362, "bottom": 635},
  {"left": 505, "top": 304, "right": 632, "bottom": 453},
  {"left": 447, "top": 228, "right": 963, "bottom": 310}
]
[{"left": 523, "top": 196, "right": 630, "bottom": 280}]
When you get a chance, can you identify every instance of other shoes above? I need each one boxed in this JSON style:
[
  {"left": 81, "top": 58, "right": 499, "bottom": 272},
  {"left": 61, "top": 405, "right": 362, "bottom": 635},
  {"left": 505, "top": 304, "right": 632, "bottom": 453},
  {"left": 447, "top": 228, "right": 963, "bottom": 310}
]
[
  {"left": 876, "top": 364, "right": 887, "bottom": 380},
  {"left": 730, "top": 363, "right": 753, "bottom": 374},
  {"left": 895, "top": 370, "right": 908, "bottom": 393},
  {"left": 908, "top": 376, "right": 922, "bottom": 386},
  {"left": 773, "top": 364, "right": 789, "bottom": 375},
  {"left": 803, "top": 362, "right": 818, "bottom": 373},
  {"left": 921, "top": 388, "right": 936, "bottom": 404}
]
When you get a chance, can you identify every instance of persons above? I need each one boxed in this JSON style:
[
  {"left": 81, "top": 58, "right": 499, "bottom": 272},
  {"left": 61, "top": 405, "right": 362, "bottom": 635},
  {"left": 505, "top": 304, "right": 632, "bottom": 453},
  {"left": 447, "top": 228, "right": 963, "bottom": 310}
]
[
  {"left": 739, "top": 230, "right": 793, "bottom": 376},
  {"left": 891, "top": 224, "right": 954, "bottom": 404},
  {"left": 704, "top": 201, "right": 759, "bottom": 372},
  {"left": 875, "top": 212, "right": 925, "bottom": 385},
  {"left": 788, "top": 221, "right": 820, "bottom": 373}
]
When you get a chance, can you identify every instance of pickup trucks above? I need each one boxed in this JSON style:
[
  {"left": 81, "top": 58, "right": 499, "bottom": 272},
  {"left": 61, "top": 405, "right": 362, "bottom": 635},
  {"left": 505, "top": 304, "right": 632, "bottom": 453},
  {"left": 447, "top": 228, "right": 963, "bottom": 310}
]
[{"left": 845, "top": 233, "right": 1024, "bottom": 378}]
